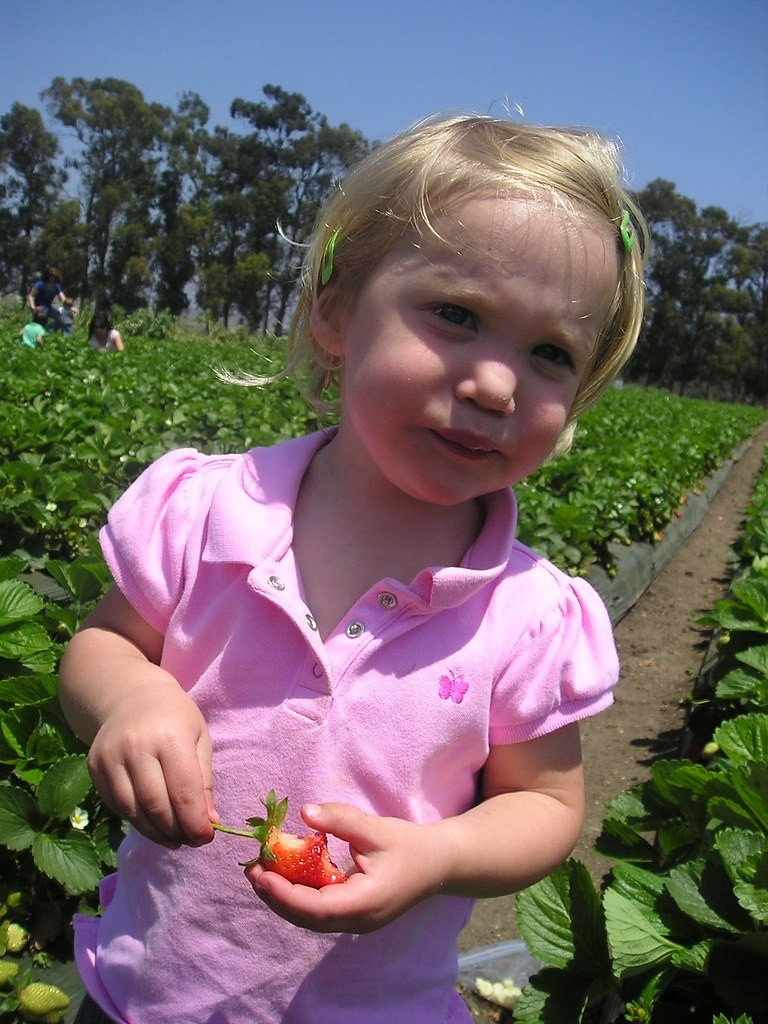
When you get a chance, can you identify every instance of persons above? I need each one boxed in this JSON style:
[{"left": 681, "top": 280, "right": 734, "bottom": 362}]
[
  {"left": 28, "top": 267, "right": 73, "bottom": 329},
  {"left": 86, "top": 312, "right": 123, "bottom": 351},
  {"left": 53, "top": 114, "right": 642, "bottom": 1024},
  {"left": 57, "top": 299, "right": 78, "bottom": 334},
  {"left": 16, "top": 310, "right": 48, "bottom": 352}
]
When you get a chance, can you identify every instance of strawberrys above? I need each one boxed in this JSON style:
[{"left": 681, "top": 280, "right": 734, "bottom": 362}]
[
  {"left": 262, "top": 826, "right": 349, "bottom": 890},
  {"left": 0, "top": 922, "right": 71, "bottom": 1024}
]
